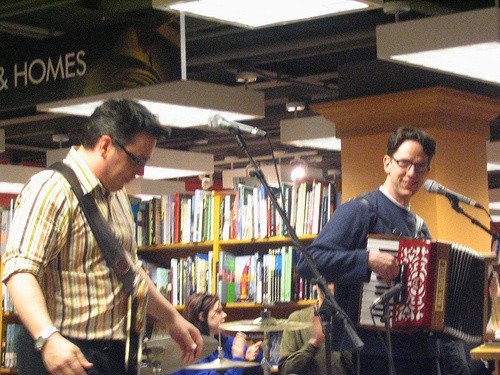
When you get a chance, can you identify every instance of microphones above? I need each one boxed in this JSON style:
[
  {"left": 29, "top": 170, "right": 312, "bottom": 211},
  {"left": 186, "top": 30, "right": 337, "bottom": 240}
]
[
  {"left": 208, "top": 114, "right": 267, "bottom": 137},
  {"left": 371, "top": 280, "right": 406, "bottom": 309},
  {"left": 423, "top": 179, "right": 484, "bottom": 209}
]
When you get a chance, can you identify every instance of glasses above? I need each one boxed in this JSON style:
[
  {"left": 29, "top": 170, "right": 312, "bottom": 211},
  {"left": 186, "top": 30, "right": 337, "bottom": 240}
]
[
  {"left": 108, "top": 133, "right": 151, "bottom": 169},
  {"left": 389, "top": 155, "right": 430, "bottom": 173}
]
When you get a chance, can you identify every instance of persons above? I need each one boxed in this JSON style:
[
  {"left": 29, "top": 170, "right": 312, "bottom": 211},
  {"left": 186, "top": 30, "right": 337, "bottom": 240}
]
[
  {"left": 166, "top": 293, "right": 264, "bottom": 375},
  {"left": 278, "top": 282, "right": 356, "bottom": 375},
  {"left": 1, "top": 98, "right": 203, "bottom": 375},
  {"left": 437, "top": 333, "right": 495, "bottom": 375},
  {"left": 297, "top": 127, "right": 497, "bottom": 375}
]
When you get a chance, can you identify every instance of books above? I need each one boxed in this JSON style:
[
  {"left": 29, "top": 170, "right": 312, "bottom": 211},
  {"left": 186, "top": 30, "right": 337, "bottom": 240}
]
[{"left": 0, "top": 179, "right": 336, "bottom": 369}]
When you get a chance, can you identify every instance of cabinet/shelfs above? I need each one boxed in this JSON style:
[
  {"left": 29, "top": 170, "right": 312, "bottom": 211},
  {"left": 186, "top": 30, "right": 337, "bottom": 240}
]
[{"left": 0, "top": 190, "right": 334, "bottom": 375}]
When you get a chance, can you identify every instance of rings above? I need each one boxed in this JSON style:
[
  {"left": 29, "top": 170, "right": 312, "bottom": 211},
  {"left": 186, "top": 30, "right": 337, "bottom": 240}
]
[{"left": 391, "top": 264, "right": 396, "bottom": 272}]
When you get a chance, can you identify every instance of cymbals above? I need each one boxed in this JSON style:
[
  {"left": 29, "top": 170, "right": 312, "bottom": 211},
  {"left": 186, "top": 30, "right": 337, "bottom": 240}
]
[
  {"left": 184, "top": 360, "right": 260, "bottom": 370},
  {"left": 219, "top": 316, "right": 312, "bottom": 332},
  {"left": 141, "top": 335, "right": 219, "bottom": 375}
]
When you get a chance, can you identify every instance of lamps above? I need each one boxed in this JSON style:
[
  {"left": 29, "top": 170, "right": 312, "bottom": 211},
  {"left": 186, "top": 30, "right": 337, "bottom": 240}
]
[{"left": 37, "top": 0, "right": 500, "bottom": 180}]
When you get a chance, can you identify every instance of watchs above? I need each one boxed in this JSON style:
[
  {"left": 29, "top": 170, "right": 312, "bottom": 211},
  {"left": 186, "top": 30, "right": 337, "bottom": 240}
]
[{"left": 34, "top": 326, "right": 60, "bottom": 351}]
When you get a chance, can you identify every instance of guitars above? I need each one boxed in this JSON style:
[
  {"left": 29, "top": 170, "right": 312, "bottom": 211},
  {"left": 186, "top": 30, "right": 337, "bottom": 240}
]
[{"left": 125, "top": 261, "right": 150, "bottom": 375}]
